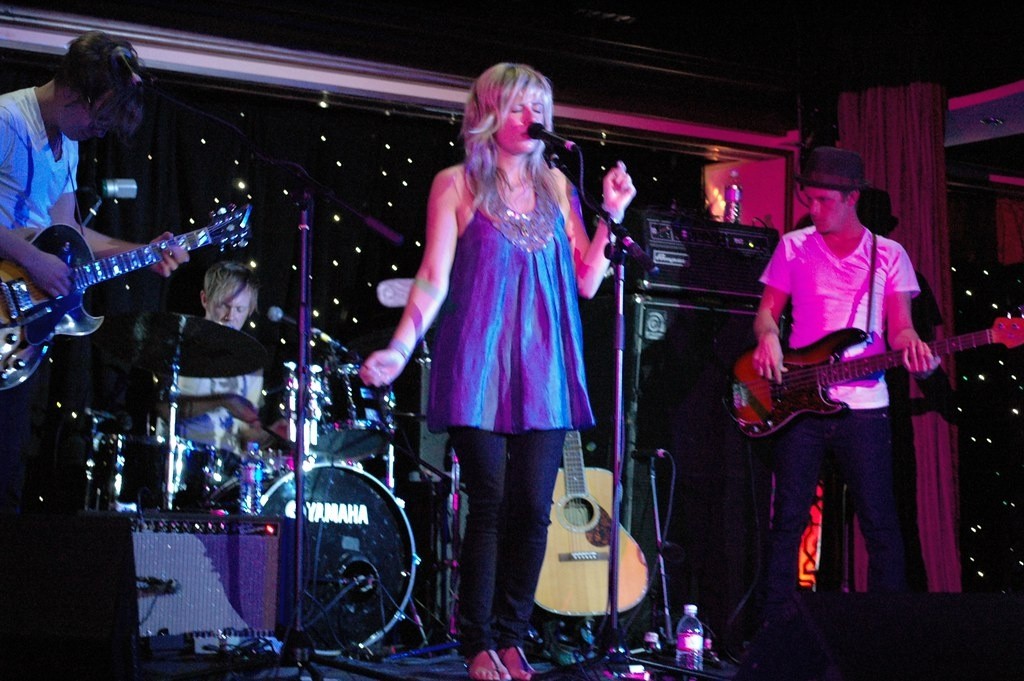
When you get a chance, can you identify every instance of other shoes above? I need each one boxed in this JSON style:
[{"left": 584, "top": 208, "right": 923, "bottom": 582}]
[
  {"left": 465, "top": 649, "right": 512, "bottom": 681},
  {"left": 497, "top": 643, "right": 536, "bottom": 681}
]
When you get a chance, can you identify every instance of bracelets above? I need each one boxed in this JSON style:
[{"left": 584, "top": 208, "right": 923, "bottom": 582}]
[
  {"left": 765, "top": 328, "right": 776, "bottom": 333},
  {"left": 601, "top": 216, "right": 620, "bottom": 225},
  {"left": 390, "top": 341, "right": 411, "bottom": 361}
]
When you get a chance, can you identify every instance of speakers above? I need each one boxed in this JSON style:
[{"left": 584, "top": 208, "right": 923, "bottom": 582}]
[
  {"left": 0, "top": 508, "right": 284, "bottom": 681},
  {"left": 581, "top": 292, "right": 772, "bottom": 661}
]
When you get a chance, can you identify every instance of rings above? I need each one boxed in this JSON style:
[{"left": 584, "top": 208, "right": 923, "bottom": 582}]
[{"left": 379, "top": 373, "right": 380, "bottom": 376}]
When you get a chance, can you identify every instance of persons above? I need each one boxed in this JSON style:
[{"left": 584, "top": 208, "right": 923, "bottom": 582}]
[
  {"left": 360, "top": 63, "right": 636, "bottom": 681},
  {"left": 754, "top": 147, "right": 942, "bottom": 622},
  {"left": 0, "top": 31, "right": 189, "bottom": 515},
  {"left": 157, "top": 263, "right": 290, "bottom": 453}
]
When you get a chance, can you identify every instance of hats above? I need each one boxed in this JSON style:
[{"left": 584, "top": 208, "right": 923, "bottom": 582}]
[{"left": 793, "top": 147, "right": 872, "bottom": 191}]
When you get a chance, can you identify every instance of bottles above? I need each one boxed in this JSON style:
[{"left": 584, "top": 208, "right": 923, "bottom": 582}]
[
  {"left": 676, "top": 604, "right": 704, "bottom": 681},
  {"left": 723, "top": 170, "right": 742, "bottom": 224},
  {"left": 239, "top": 442, "right": 262, "bottom": 517}
]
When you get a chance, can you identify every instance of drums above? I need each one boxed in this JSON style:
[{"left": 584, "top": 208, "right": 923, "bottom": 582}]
[
  {"left": 100, "top": 432, "right": 217, "bottom": 510},
  {"left": 282, "top": 360, "right": 397, "bottom": 460},
  {"left": 209, "top": 458, "right": 419, "bottom": 656}
]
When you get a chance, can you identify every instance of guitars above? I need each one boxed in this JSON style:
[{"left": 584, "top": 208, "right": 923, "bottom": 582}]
[
  {"left": 533, "top": 431, "right": 649, "bottom": 618},
  {"left": 732, "top": 306, "right": 1024, "bottom": 439},
  {"left": 1, "top": 192, "right": 254, "bottom": 392}
]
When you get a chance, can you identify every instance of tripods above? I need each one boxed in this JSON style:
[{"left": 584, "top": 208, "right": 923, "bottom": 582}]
[
  {"left": 534, "top": 155, "right": 727, "bottom": 681},
  {"left": 142, "top": 80, "right": 409, "bottom": 681}
]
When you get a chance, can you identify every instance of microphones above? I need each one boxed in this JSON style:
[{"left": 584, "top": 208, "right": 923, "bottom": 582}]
[
  {"left": 268, "top": 307, "right": 321, "bottom": 335},
  {"left": 112, "top": 45, "right": 144, "bottom": 87},
  {"left": 166, "top": 579, "right": 178, "bottom": 593},
  {"left": 357, "top": 575, "right": 379, "bottom": 586},
  {"left": 526, "top": 123, "right": 578, "bottom": 152},
  {"left": 631, "top": 449, "right": 670, "bottom": 459}
]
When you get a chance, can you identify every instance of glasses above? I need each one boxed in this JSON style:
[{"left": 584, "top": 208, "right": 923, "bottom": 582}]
[{"left": 85, "top": 97, "right": 123, "bottom": 136}]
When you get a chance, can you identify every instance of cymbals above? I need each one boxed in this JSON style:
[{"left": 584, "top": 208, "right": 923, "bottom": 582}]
[{"left": 100, "top": 308, "right": 265, "bottom": 379}]
